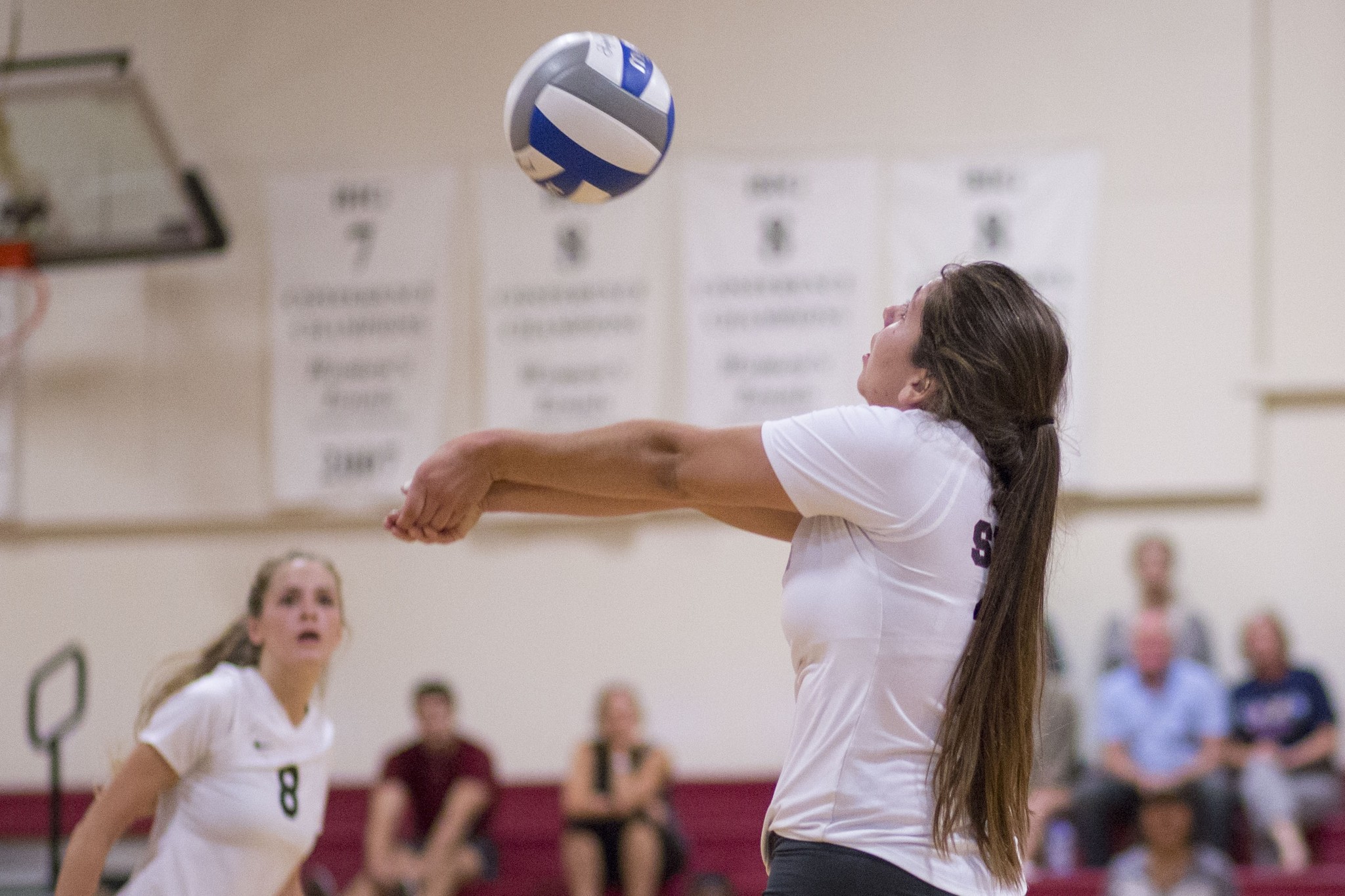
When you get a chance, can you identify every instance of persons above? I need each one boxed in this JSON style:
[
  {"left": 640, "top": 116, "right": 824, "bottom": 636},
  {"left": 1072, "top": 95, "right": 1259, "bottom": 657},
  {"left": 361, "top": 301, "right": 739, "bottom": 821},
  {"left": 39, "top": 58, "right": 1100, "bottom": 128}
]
[
  {"left": 45, "top": 548, "right": 345, "bottom": 895},
  {"left": 343, "top": 683, "right": 501, "bottom": 896},
  {"left": 1019, "top": 536, "right": 1345, "bottom": 896},
  {"left": 382, "top": 259, "right": 1069, "bottom": 896},
  {"left": 556, "top": 685, "right": 688, "bottom": 895}
]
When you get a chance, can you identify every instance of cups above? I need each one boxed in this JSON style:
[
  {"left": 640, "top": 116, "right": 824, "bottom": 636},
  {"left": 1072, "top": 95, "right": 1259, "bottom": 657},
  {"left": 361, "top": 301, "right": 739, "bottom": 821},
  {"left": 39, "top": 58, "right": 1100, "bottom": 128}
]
[{"left": 1044, "top": 822, "right": 1077, "bottom": 874}]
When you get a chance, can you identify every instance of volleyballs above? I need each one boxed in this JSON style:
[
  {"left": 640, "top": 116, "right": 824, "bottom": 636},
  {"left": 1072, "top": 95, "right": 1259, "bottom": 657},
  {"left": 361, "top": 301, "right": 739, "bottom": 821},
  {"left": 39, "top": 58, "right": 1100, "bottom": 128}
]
[{"left": 503, "top": 31, "right": 675, "bottom": 206}]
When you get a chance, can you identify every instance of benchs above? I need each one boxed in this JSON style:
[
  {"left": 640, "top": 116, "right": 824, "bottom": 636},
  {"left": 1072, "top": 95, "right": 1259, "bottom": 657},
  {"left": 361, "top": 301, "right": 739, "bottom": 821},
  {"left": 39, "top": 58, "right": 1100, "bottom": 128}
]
[{"left": 0, "top": 776, "right": 1345, "bottom": 896}]
